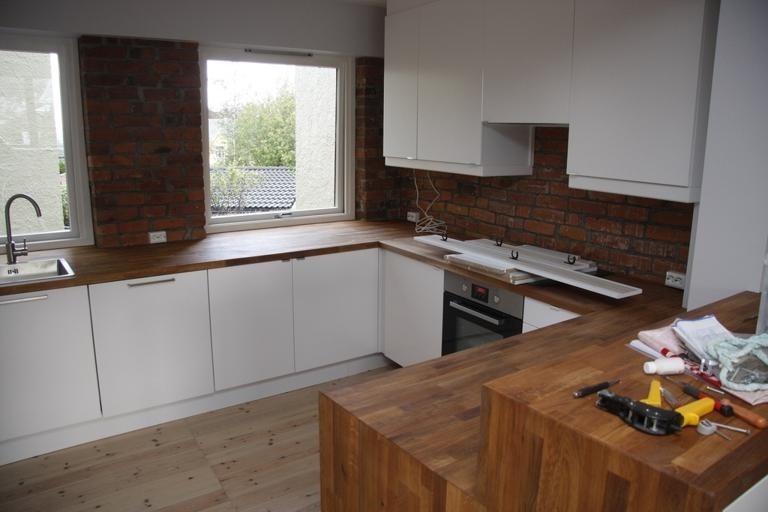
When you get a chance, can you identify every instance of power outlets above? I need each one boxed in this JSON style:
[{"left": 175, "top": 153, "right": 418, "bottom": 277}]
[{"left": 407, "top": 211, "right": 419, "bottom": 222}]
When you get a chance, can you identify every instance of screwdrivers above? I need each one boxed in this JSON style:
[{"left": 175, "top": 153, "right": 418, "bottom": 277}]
[
  {"left": 666, "top": 376, "right": 733, "bottom": 417},
  {"left": 661, "top": 388, "right": 684, "bottom": 408},
  {"left": 708, "top": 392, "right": 766, "bottom": 429}
]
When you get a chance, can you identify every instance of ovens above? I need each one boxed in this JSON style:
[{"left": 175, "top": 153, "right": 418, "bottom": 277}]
[{"left": 442, "top": 269, "right": 524, "bottom": 354}]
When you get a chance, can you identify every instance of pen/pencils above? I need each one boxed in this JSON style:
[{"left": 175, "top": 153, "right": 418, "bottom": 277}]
[{"left": 573, "top": 380, "right": 620, "bottom": 399}]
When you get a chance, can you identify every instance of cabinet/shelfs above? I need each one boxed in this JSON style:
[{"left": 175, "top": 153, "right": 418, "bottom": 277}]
[
  {"left": 1, "top": 285, "right": 101, "bottom": 444},
  {"left": 208, "top": 247, "right": 379, "bottom": 397},
  {"left": 382, "top": 0, "right": 536, "bottom": 178},
  {"left": 566, "top": 0, "right": 721, "bottom": 204},
  {"left": 481, "top": 0, "right": 573, "bottom": 125},
  {"left": 87, "top": 268, "right": 216, "bottom": 419},
  {"left": 522, "top": 295, "right": 581, "bottom": 334},
  {"left": 379, "top": 247, "right": 444, "bottom": 368}
]
